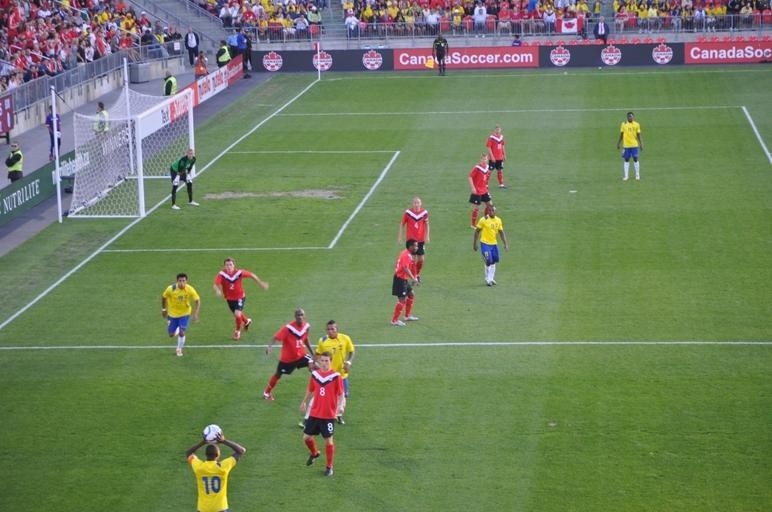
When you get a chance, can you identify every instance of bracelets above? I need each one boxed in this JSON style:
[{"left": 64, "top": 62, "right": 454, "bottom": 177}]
[{"left": 162, "top": 308, "right": 166, "bottom": 312}]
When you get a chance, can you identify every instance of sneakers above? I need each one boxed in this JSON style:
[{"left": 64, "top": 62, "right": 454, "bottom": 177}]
[
  {"left": 470, "top": 223, "right": 477, "bottom": 230},
  {"left": 409, "top": 276, "right": 420, "bottom": 286},
  {"left": 263, "top": 391, "right": 275, "bottom": 403},
  {"left": 306, "top": 451, "right": 321, "bottom": 466},
  {"left": 336, "top": 415, "right": 345, "bottom": 425},
  {"left": 244, "top": 318, "right": 252, "bottom": 327},
  {"left": 188, "top": 200, "right": 200, "bottom": 206},
  {"left": 390, "top": 315, "right": 419, "bottom": 328},
  {"left": 486, "top": 280, "right": 498, "bottom": 287},
  {"left": 325, "top": 465, "right": 333, "bottom": 476},
  {"left": 171, "top": 204, "right": 181, "bottom": 210},
  {"left": 498, "top": 185, "right": 508, "bottom": 190},
  {"left": 232, "top": 329, "right": 242, "bottom": 340},
  {"left": 176, "top": 347, "right": 184, "bottom": 357},
  {"left": 298, "top": 419, "right": 308, "bottom": 427},
  {"left": 622, "top": 174, "right": 642, "bottom": 181}
]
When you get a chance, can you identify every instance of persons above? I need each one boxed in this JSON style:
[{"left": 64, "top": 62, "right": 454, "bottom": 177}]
[
  {"left": 185, "top": 432, "right": 246, "bottom": 512},
  {"left": 92, "top": 102, "right": 110, "bottom": 135},
  {"left": 169, "top": 148, "right": 199, "bottom": 210},
  {"left": 262, "top": 308, "right": 355, "bottom": 475},
  {"left": 213, "top": 258, "right": 268, "bottom": 340},
  {"left": 390, "top": 238, "right": 420, "bottom": 328},
  {"left": 468, "top": 127, "right": 508, "bottom": 287},
  {"left": 161, "top": 273, "right": 200, "bottom": 357},
  {"left": 45, "top": 105, "right": 62, "bottom": 161},
  {"left": 398, "top": 197, "right": 429, "bottom": 286},
  {"left": 5, "top": 141, "right": 23, "bottom": 183},
  {"left": 617, "top": 113, "right": 642, "bottom": 181}
]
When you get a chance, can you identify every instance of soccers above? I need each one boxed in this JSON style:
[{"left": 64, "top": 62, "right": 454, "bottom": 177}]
[{"left": 202, "top": 425, "right": 222, "bottom": 443}]
